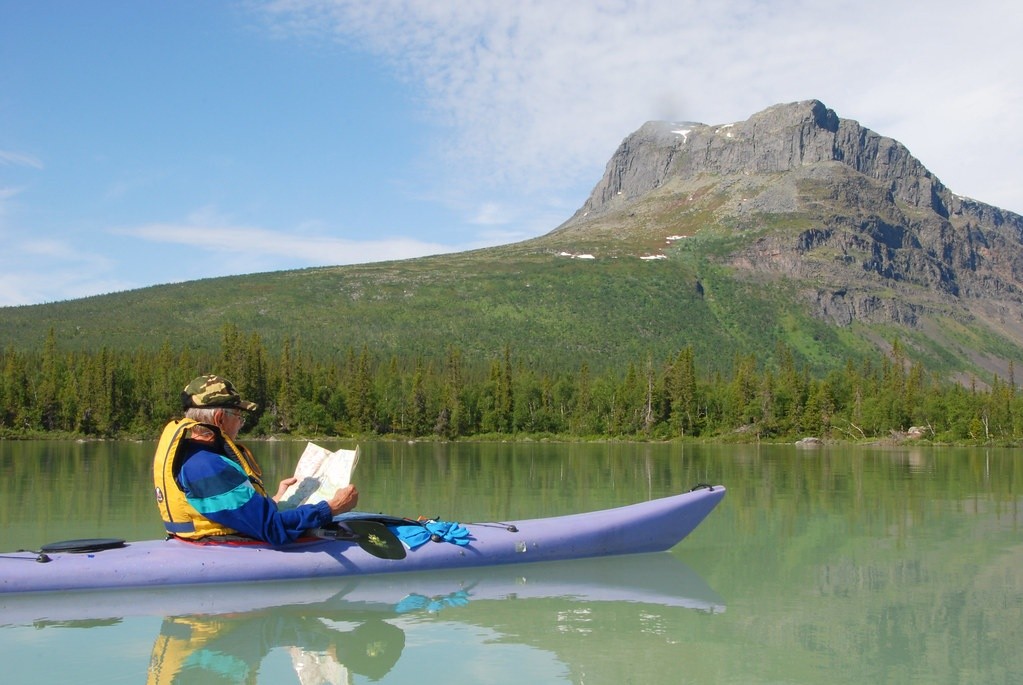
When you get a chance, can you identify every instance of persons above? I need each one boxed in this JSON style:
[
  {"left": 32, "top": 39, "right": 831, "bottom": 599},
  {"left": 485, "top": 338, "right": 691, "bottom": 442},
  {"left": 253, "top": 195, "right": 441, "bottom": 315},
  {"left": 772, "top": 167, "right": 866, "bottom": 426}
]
[{"left": 152, "top": 373, "right": 360, "bottom": 550}]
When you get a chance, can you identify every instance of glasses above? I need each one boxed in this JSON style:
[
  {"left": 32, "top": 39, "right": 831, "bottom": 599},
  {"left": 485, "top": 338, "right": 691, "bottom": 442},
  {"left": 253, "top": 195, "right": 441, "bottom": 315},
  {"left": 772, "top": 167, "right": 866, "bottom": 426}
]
[{"left": 225, "top": 410, "right": 248, "bottom": 423}]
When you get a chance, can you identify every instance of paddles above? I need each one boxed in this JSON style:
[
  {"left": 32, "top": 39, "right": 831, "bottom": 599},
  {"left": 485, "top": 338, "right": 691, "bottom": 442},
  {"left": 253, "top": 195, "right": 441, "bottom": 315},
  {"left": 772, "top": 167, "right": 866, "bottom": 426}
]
[{"left": 320, "top": 518, "right": 407, "bottom": 561}]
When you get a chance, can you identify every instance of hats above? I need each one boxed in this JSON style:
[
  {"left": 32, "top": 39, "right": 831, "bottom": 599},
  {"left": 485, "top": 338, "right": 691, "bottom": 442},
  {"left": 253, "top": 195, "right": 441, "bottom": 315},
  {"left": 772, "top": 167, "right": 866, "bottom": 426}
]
[{"left": 181, "top": 373, "right": 259, "bottom": 411}]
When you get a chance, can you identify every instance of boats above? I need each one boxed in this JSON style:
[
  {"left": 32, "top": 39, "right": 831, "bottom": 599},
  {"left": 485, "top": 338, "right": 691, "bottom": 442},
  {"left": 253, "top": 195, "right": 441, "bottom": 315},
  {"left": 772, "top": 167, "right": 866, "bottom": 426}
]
[
  {"left": 0, "top": 483, "right": 726, "bottom": 592},
  {"left": 2, "top": 551, "right": 726, "bottom": 615}
]
